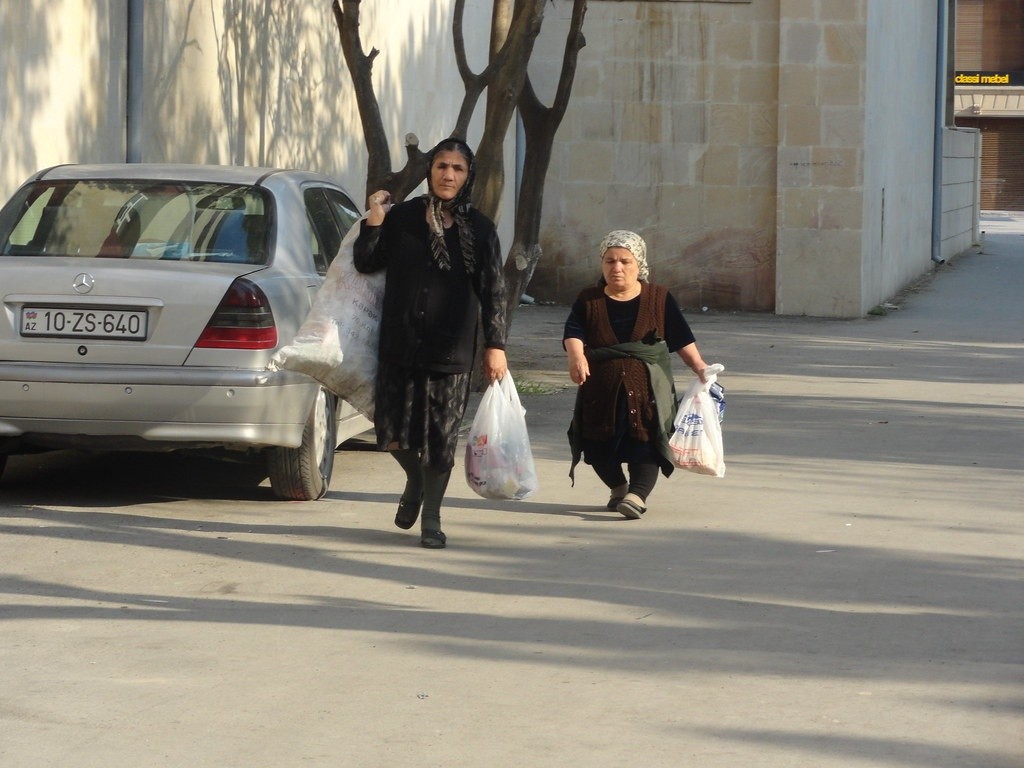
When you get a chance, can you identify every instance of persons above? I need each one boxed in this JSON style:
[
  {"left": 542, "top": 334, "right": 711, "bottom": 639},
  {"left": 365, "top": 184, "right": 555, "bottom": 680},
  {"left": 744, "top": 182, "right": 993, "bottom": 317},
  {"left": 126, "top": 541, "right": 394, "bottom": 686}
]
[
  {"left": 353, "top": 138, "right": 508, "bottom": 549},
  {"left": 562, "top": 229, "right": 709, "bottom": 519}
]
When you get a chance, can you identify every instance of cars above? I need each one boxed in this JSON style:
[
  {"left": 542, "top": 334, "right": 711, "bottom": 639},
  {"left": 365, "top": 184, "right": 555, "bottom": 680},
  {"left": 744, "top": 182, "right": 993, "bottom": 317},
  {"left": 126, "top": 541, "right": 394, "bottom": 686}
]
[{"left": 0, "top": 163, "right": 375, "bottom": 503}]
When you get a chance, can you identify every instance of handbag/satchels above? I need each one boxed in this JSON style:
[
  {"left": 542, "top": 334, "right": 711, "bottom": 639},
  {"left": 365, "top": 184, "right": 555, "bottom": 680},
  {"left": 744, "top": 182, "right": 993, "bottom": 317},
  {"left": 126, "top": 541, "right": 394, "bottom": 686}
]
[
  {"left": 665, "top": 362, "right": 725, "bottom": 477},
  {"left": 465, "top": 369, "right": 537, "bottom": 499}
]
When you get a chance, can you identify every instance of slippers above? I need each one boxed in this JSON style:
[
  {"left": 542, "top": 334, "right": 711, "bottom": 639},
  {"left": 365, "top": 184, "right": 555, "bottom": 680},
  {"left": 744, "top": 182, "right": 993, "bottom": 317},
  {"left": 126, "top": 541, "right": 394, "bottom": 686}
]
[
  {"left": 394, "top": 491, "right": 424, "bottom": 530},
  {"left": 421, "top": 530, "right": 447, "bottom": 547}
]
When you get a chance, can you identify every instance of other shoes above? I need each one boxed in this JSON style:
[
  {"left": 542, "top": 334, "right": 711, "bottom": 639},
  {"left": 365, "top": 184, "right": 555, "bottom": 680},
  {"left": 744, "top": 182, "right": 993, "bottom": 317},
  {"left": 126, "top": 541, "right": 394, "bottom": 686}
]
[
  {"left": 617, "top": 494, "right": 648, "bottom": 518},
  {"left": 608, "top": 486, "right": 629, "bottom": 511}
]
